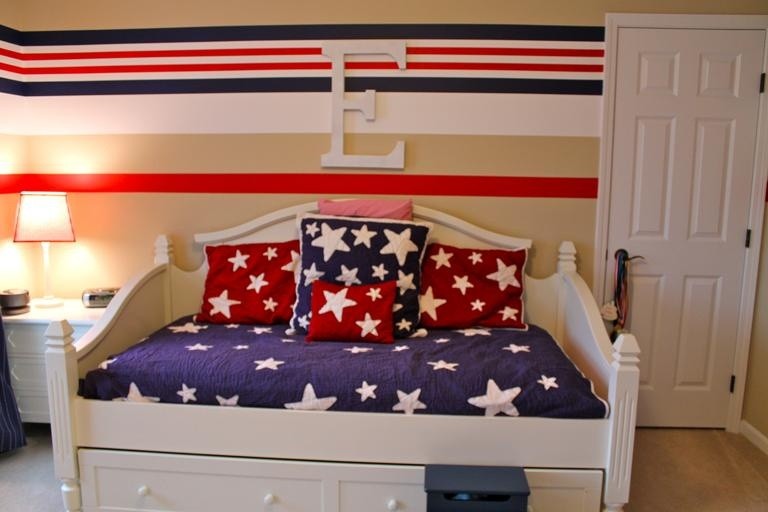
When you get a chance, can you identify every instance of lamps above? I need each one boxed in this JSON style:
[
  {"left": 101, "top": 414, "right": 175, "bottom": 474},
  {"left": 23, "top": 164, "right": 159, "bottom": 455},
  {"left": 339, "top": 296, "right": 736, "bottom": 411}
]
[{"left": 12, "top": 188, "right": 75, "bottom": 309}]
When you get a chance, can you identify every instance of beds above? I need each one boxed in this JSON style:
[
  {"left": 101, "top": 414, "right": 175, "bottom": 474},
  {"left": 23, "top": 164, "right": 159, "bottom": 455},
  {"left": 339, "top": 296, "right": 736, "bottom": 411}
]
[{"left": 42, "top": 201, "right": 642, "bottom": 511}]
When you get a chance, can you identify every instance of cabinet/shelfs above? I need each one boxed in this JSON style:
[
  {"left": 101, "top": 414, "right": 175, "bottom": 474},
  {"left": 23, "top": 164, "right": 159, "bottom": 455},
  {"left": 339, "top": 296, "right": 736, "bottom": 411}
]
[{"left": 76, "top": 444, "right": 607, "bottom": 512}]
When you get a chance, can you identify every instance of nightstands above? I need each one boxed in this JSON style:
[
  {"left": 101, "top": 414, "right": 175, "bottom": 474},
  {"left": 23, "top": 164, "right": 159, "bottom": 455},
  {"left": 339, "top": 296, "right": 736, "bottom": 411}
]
[{"left": 1, "top": 297, "right": 108, "bottom": 432}]
